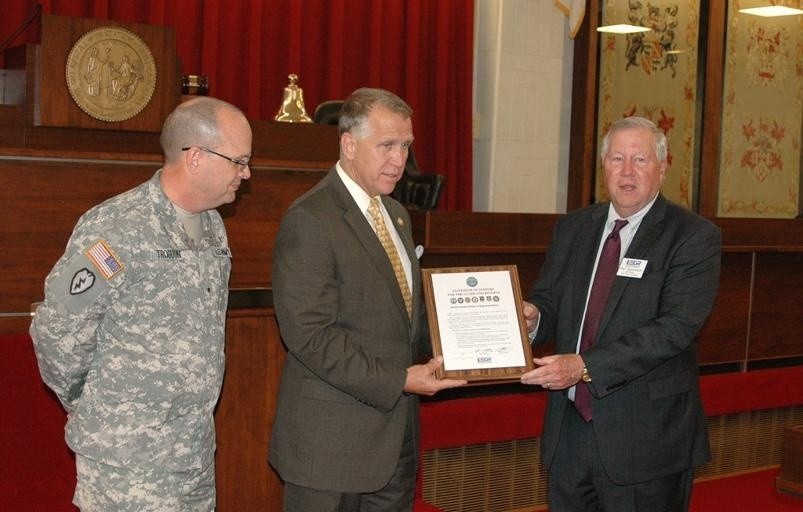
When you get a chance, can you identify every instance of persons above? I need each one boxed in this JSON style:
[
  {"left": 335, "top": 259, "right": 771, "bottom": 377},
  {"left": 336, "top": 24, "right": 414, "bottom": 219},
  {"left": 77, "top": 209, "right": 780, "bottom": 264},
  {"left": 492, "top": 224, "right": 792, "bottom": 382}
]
[
  {"left": 87, "top": 48, "right": 136, "bottom": 101},
  {"left": 28, "top": 96, "right": 254, "bottom": 512},
  {"left": 267, "top": 86, "right": 468, "bottom": 512},
  {"left": 523, "top": 117, "right": 725, "bottom": 512}
]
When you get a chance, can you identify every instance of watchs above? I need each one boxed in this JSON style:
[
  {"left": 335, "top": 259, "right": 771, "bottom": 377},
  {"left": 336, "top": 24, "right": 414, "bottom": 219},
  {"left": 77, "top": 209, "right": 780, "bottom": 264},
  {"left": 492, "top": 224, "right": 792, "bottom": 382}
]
[{"left": 581, "top": 362, "right": 593, "bottom": 385}]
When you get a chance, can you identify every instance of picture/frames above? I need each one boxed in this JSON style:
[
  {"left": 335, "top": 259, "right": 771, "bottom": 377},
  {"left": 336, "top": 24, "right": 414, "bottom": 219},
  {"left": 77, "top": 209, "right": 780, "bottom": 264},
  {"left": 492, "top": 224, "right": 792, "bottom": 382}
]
[{"left": 422, "top": 265, "right": 536, "bottom": 387}]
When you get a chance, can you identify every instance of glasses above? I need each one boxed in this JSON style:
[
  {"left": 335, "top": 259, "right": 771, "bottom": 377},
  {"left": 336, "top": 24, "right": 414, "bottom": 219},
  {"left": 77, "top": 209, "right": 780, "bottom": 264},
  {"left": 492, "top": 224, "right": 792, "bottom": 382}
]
[{"left": 182, "top": 147, "right": 251, "bottom": 170}]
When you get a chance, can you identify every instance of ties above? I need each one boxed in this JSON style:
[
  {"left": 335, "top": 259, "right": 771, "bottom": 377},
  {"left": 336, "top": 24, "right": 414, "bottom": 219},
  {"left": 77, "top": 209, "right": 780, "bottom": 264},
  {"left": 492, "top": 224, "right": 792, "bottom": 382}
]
[
  {"left": 367, "top": 198, "right": 412, "bottom": 329},
  {"left": 574, "top": 219, "right": 629, "bottom": 421}
]
